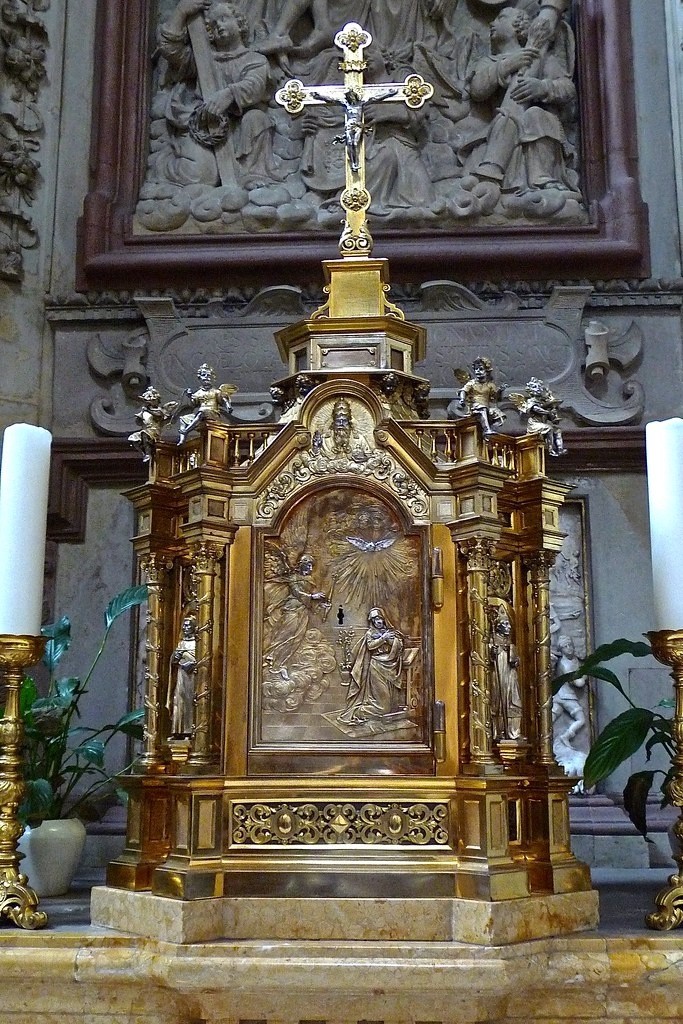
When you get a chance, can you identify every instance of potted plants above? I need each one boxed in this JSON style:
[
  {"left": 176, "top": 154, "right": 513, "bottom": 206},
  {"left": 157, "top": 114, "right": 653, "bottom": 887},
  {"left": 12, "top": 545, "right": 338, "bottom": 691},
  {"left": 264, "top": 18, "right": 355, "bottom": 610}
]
[
  {"left": 551, "top": 640, "right": 681, "bottom": 871},
  {"left": 1, "top": 586, "right": 148, "bottom": 898}
]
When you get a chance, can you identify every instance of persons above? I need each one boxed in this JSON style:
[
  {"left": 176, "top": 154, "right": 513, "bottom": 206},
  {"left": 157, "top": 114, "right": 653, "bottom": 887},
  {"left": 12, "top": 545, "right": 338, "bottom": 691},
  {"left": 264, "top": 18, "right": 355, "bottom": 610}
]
[
  {"left": 266, "top": 555, "right": 326, "bottom": 680},
  {"left": 168, "top": 617, "right": 197, "bottom": 740},
  {"left": 337, "top": 607, "right": 405, "bottom": 726},
  {"left": 162, "top": 0, "right": 283, "bottom": 188},
  {"left": 524, "top": 378, "right": 568, "bottom": 459},
  {"left": 292, "top": 39, "right": 432, "bottom": 206},
  {"left": 490, "top": 605, "right": 522, "bottom": 740},
  {"left": 129, "top": 386, "right": 176, "bottom": 464},
  {"left": 331, "top": 396, "right": 352, "bottom": 453},
  {"left": 474, "top": 8, "right": 576, "bottom": 191},
  {"left": 458, "top": 357, "right": 506, "bottom": 437},
  {"left": 178, "top": 364, "right": 239, "bottom": 445},
  {"left": 553, "top": 636, "right": 589, "bottom": 747}
]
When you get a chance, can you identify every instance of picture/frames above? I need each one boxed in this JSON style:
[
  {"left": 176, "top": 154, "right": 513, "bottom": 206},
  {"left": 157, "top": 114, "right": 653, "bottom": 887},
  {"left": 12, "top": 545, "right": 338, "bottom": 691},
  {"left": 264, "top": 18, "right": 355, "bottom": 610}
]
[{"left": 77, "top": 2, "right": 651, "bottom": 283}]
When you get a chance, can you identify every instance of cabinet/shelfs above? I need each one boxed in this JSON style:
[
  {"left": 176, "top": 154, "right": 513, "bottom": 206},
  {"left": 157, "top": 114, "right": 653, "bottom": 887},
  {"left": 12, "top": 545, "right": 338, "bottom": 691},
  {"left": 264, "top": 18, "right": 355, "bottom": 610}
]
[{"left": 0, "top": 876, "right": 682, "bottom": 1024}]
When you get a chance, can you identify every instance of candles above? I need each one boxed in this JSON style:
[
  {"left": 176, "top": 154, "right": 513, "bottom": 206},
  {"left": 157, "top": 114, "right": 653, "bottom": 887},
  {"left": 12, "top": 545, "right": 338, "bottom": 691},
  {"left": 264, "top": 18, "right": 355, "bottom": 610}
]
[
  {"left": 0, "top": 424, "right": 52, "bottom": 636},
  {"left": 645, "top": 417, "right": 683, "bottom": 634}
]
